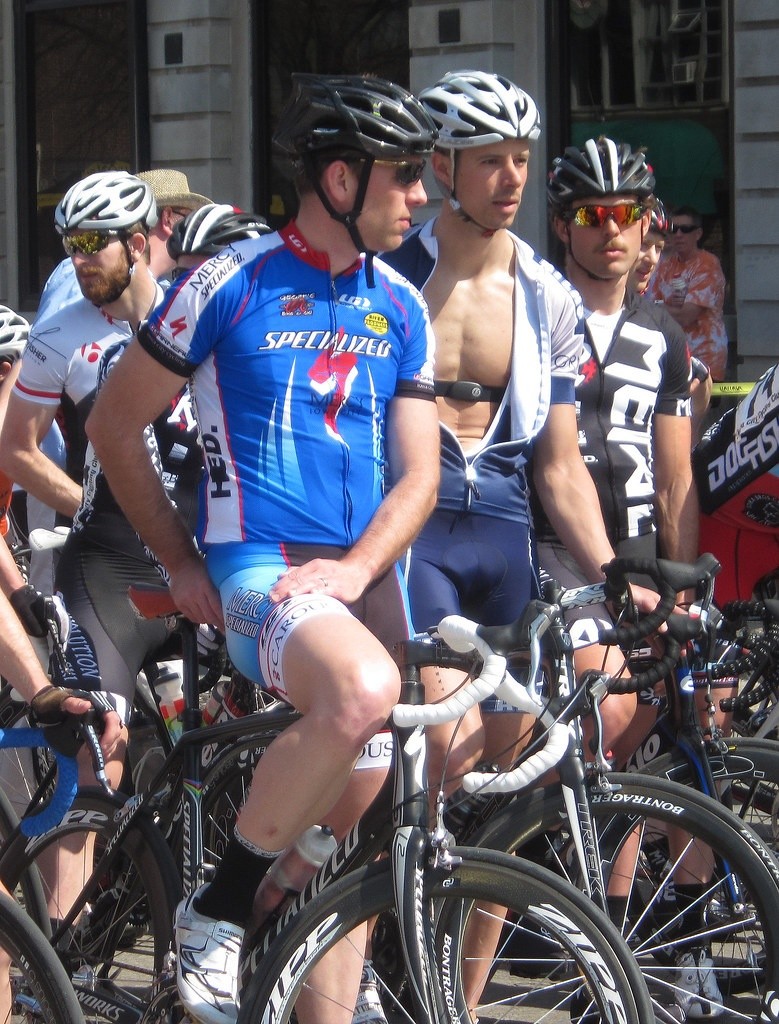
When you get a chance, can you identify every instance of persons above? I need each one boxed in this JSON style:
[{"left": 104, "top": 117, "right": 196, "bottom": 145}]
[
  {"left": 538, "top": 135, "right": 738, "bottom": 1024},
  {"left": 85, "top": 70, "right": 700, "bottom": 1024},
  {"left": 0, "top": 169, "right": 263, "bottom": 1024},
  {"left": 652, "top": 207, "right": 727, "bottom": 409}
]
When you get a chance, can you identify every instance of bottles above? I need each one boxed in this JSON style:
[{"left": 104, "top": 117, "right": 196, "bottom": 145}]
[
  {"left": 152, "top": 667, "right": 185, "bottom": 747},
  {"left": 443, "top": 762, "right": 502, "bottom": 833},
  {"left": 244, "top": 825, "right": 338, "bottom": 942},
  {"left": 200, "top": 680, "right": 231, "bottom": 726}
]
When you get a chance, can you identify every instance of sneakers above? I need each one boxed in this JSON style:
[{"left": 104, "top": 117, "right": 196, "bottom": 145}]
[
  {"left": 175, "top": 883, "right": 245, "bottom": 1024},
  {"left": 352, "top": 960, "right": 388, "bottom": 1024},
  {"left": 675, "top": 949, "right": 724, "bottom": 1017}
]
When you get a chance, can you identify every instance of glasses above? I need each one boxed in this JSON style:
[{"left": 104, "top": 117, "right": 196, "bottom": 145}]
[
  {"left": 62, "top": 229, "right": 131, "bottom": 256},
  {"left": 668, "top": 225, "right": 702, "bottom": 234},
  {"left": 565, "top": 204, "right": 651, "bottom": 226},
  {"left": 358, "top": 158, "right": 427, "bottom": 185}
]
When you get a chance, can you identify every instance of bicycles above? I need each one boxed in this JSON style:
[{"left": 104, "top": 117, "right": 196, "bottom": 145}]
[{"left": 0, "top": 552, "right": 778, "bottom": 1024}]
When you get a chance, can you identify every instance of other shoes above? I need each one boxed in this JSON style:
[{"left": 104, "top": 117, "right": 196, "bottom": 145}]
[
  {"left": 570, "top": 985, "right": 685, "bottom": 1024},
  {"left": 657, "top": 901, "right": 743, "bottom": 933}
]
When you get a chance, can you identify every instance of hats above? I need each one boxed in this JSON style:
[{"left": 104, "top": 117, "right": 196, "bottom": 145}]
[{"left": 132, "top": 169, "right": 215, "bottom": 210}]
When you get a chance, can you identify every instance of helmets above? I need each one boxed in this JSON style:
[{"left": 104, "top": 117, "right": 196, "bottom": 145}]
[
  {"left": 545, "top": 138, "right": 656, "bottom": 206},
  {"left": 0, "top": 305, "right": 31, "bottom": 358},
  {"left": 166, "top": 204, "right": 275, "bottom": 261},
  {"left": 418, "top": 71, "right": 542, "bottom": 148},
  {"left": 270, "top": 72, "right": 440, "bottom": 181},
  {"left": 54, "top": 171, "right": 158, "bottom": 234},
  {"left": 649, "top": 198, "right": 673, "bottom": 237}
]
